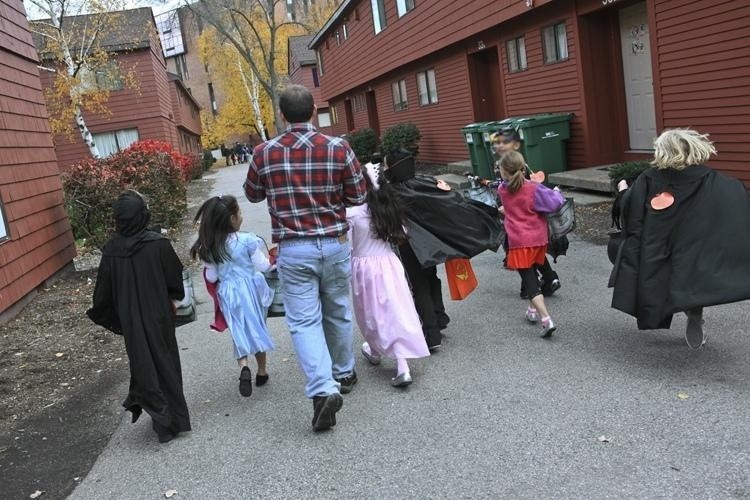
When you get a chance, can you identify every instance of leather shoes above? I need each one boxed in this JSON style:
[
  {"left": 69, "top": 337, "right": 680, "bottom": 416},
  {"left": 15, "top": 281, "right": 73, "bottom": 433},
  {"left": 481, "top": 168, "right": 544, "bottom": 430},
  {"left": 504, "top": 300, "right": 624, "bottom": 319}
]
[
  {"left": 391, "top": 372, "right": 412, "bottom": 386},
  {"left": 540, "top": 319, "right": 555, "bottom": 337},
  {"left": 362, "top": 341, "right": 380, "bottom": 365},
  {"left": 525, "top": 306, "right": 537, "bottom": 322}
]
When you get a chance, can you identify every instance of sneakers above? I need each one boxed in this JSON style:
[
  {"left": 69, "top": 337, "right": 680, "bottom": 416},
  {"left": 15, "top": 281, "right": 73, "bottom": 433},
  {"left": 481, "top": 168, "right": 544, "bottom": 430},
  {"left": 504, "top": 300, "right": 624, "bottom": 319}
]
[
  {"left": 335, "top": 369, "right": 357, "bottom": 394},
  {"left": 684, "top": 308, "right": 704, "bottom": 352},
  {"left": 312, "top": 393, "right": 343, "bottom": 429},
  {"left": 239, "top": 366, "right": 252, "bottom": 397},
  {"left": 256, "top": 373, "right": 268, "bottom": 386},
  {"left": 543, "top": 272, "right": 561, "bottom": 296},
  {"left": 157, "top": 429, "right": 174, "bottom": 442}
]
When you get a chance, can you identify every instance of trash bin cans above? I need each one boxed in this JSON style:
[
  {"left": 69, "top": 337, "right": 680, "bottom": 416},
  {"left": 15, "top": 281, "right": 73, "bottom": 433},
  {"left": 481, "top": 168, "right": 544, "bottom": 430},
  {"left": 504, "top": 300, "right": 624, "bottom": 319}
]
[{"left": 459, "top": 111, "right": 576, "bottom": 180}]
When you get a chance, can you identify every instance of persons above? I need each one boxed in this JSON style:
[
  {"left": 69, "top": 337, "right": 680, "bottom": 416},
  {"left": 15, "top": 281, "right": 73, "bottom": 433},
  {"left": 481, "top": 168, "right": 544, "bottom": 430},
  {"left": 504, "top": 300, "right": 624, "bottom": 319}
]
[
  {"left": 243, "top": 84, "right": 367, "bottom": 433},
  {"left": 345, "top": 166, "right": 431, "bottom": 387},
  {"left": 189, "top": 193, "right": 275, "bottom": 397},
  {"left": 497, "top": 149, "right": 564, "bottom": 338},
  {"left": 220, "top": 142, "right": 253, "bottom": 166},
  {"left": 606, "top": 127, "right": 750, "bottom": 351},
  {"left": 487, "top": 127, "right": 562, "bottom": 300},
  {"left": 86, "top": 189, "right": 192, "bottom": 443},
  {"left": 381, "top": 148, "right": 503, "bottom": 349}
]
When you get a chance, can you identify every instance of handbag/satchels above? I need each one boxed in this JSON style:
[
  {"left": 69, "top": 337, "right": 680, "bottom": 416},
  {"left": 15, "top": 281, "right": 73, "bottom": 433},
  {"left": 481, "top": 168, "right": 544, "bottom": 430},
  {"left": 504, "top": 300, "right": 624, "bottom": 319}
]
[{"left": 445, "top": 257, "right": 478, "bottom": 300}]
